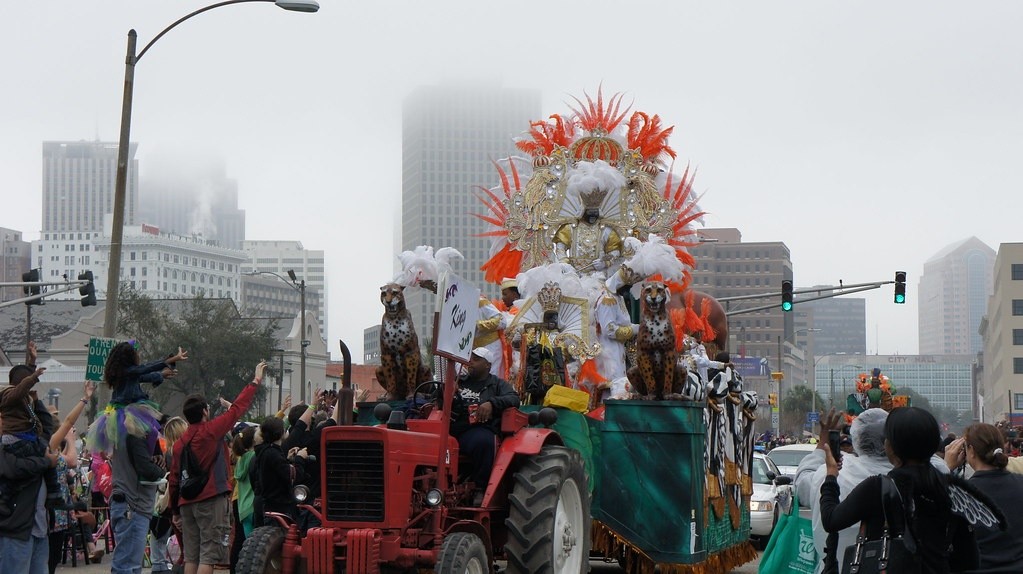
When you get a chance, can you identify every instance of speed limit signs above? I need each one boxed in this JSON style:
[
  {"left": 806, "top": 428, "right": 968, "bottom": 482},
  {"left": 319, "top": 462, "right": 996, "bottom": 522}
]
[{"left": 772, "top": 417, "right": 779, "bottom": 428}]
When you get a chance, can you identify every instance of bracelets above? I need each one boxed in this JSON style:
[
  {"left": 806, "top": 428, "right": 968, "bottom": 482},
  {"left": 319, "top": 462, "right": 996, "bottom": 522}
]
[
  {"left": 80, "top": 398, "right": 88, "bottom": 403},
  {"left": 254, "top": 376, "right": 260, "bottom": 384},
  {"left": 309, "top": 404, "right": 316, "bottom": 411}
]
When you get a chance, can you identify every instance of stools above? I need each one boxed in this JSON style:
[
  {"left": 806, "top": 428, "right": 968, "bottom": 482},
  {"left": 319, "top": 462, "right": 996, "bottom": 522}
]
[
  {"left": 89, "top": 506, "right": 115, "bottom": 554},
  {"left": 61, "top": 514, "right": 89, "bottom": 567}
]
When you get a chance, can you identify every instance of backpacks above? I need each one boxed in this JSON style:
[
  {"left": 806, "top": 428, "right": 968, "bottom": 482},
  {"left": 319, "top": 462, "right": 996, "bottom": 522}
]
[{"left": 177, "top": 431, "right": 212, "bottom": 500}]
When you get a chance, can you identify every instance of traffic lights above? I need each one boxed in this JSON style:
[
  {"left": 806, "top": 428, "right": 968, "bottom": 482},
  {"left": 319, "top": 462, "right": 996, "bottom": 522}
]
[
  {"left": 780, "top": 279, "right": 794, "bottom": 312},
  {"left": 78, "top": 271, "right": 98, "bottom": 307},
  {"left": 894, "top": 271, "right": 907, "bottom": 304},
  {"left": 768, "top": 394, "right": 777, "bottom": 405},
  {"left": 22, "top": 269, "right": 42, "bottom": 306}
]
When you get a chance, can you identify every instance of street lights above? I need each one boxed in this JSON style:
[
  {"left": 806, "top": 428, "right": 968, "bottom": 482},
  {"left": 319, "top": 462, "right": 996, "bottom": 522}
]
[
  {"left": 842, "top": 374, "right": 860, "bottom": 402},
  {"left": 810, "top": 351, "right": 846, "bottom": 438},
  {"left": 777, "top": 327, "right": 823, "bottom": 438},
  {"left": 268, "top": 348, "right": 286, "bottom": 413},
  {"left": 829, "top": 365, "right": 862, "bottom": 403},
  {"left": 95, "top": 0, "right": 323, "bottom": 428},
  {"left": 239, "top": 267, "right": 312, "bottom": 405}
]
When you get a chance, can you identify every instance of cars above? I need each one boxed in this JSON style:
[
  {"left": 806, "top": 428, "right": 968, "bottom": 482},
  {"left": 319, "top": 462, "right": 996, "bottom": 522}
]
[
  {"left": 767, "top": 443, "right": 820, "bottom": 482},
  {"left": 747, "top": 453, "right": 793, "bottom": 549}
]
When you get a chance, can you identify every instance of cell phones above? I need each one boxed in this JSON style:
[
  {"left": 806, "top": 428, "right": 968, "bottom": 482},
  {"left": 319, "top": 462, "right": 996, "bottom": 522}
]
[{"left": 828, "top": 429, "right": 841, "bottom": 462}]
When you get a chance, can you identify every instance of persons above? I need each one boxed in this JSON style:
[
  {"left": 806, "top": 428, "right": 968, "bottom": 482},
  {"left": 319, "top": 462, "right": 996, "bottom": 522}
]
[
  {"left": 552, "top": 185, "right": 622, "bottom": 277},
  {"left": 504, "top": 280, "right": 579, "bottom": 387},
  {"left": 471, "top": 292, "right": 506, "bottom": 380},
  {"left": 689, "top": 330, "right": 736, "bottom": 387},
  {"left": 755, "top": 430, "right": 817, "bottom": 450},
  {"left": 862, "top": 368, "right": 885, "bottom": 408},
  {"left": 500, "top": 278, "right": 531, "bottom": 394},
  {"left": 706, "top": 353, "right": 758, "bottom": 421},
  {"left": 0, "top": 341, "right": 104, "bottom": 574},
  {"left": 793, "top": 406, "right": 1023, "bottom": 574},
  {"left": 428, "top": 346, "right": 520, "bottom": 508},
  {"left": 104, "top": 339, "right": 371, "bottom": 574}
]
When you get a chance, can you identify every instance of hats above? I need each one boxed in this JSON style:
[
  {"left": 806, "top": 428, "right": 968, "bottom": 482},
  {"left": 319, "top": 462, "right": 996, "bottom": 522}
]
[
  {"left": 472, "top": 347, "right": 493, "bottom": 364},
  {"left": 501, "top": 277, "right": 518, "bottom": 291}
]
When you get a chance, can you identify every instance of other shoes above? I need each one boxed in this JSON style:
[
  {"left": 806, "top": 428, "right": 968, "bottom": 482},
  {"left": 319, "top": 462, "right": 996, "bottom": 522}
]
[
  {"left": 45, "top": 494, "right": 75, "bottom": 511},
  {"left": 91, "top": 549, "right": 105, "bottom": 563}
]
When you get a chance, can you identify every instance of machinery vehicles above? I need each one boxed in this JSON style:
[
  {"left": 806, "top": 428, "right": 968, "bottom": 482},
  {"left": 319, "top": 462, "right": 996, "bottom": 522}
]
[{"left": 232, "top": 288, "right": 755, "bottom": 574}]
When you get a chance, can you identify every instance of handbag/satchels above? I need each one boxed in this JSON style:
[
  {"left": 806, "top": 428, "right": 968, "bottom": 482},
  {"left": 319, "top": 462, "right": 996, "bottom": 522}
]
[
  {"left": 757, "top": 487, "right": 820, "bottom": 574},
  {"left": 839, "top": 476, "right": 908, "bottom": 574}
]
